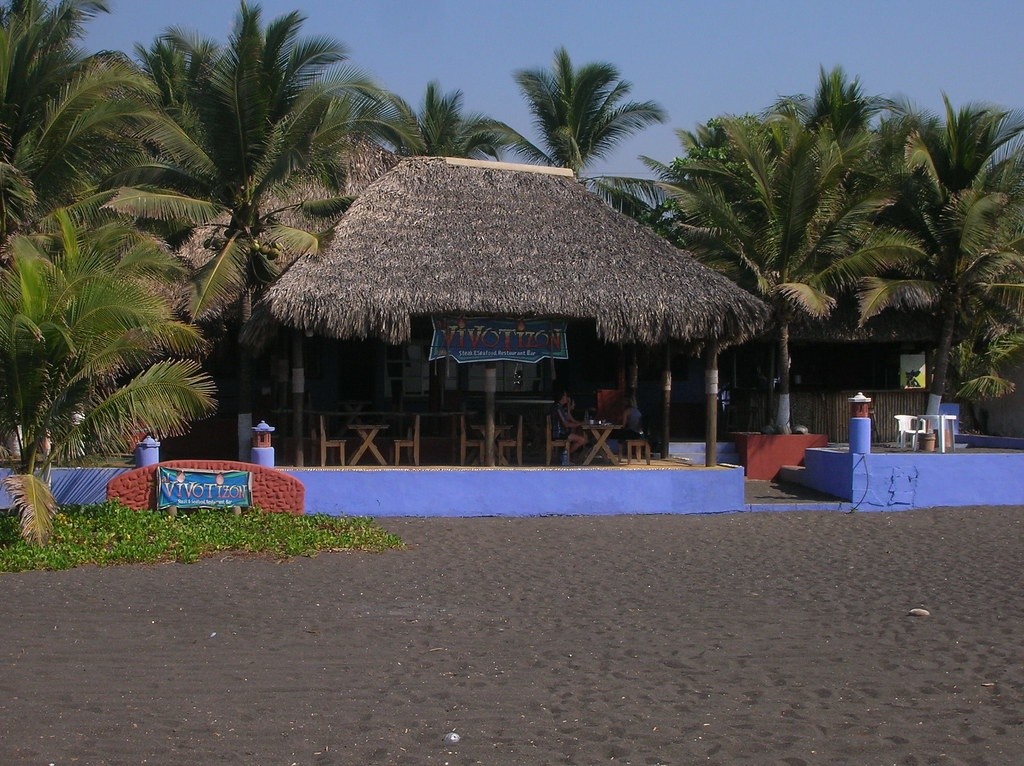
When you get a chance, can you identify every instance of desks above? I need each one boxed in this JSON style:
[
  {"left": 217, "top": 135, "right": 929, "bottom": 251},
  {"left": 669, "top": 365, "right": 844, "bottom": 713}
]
[
  {"left": 913, "top": 415, "right": 956, "bottom": 453},
  {"left": 469, "top": 424, "right": 513, "bottom": 466},
  {"left": 345, "top": 424, "right": 390, "bottom": 466},
  {"left": 577, "top": 424, "right": 623, "bottom": 465}
]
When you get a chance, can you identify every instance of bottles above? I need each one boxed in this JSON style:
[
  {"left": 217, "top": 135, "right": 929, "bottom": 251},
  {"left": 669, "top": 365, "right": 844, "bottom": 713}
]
[{"left": 562, "top": 448, "right": 568, "bottom": 465}]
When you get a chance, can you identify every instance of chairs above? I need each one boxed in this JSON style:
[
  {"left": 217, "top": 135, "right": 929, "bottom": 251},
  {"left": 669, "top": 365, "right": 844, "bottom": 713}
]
[
  {"left": 309, "top": 414, "right": 347, "bottom": 467},
  {"left": 894, "top": 414, "right": 926, "bottom": 449},
  {"left": 451, "top": 415, "right": 484, "bottom": 466},
  {"left": 618, "top": 425, "right": 651, "bottom": 465},
  {"left": 389, "top": 414, "right": 419, "bottom": 466},
  {"left": 545, "top": 415, "right": 572, "bottom": 465},
  {"left": 495, "top": 414, "right": 522, "bottom": 466}
]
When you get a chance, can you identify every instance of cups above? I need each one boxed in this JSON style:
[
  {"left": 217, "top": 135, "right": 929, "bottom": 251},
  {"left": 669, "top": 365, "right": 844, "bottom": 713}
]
[{"left": 589, "top": 420, "right": 594, "bottom": 424}]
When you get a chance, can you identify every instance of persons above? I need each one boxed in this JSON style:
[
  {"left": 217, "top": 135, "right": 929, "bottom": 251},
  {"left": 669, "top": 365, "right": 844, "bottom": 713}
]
[
  {"left": 615, "top": 395, "right": 642, "bottom": 461},
  {"left": 551, "top": 390, "right": 586, "bottom": 465}
]
